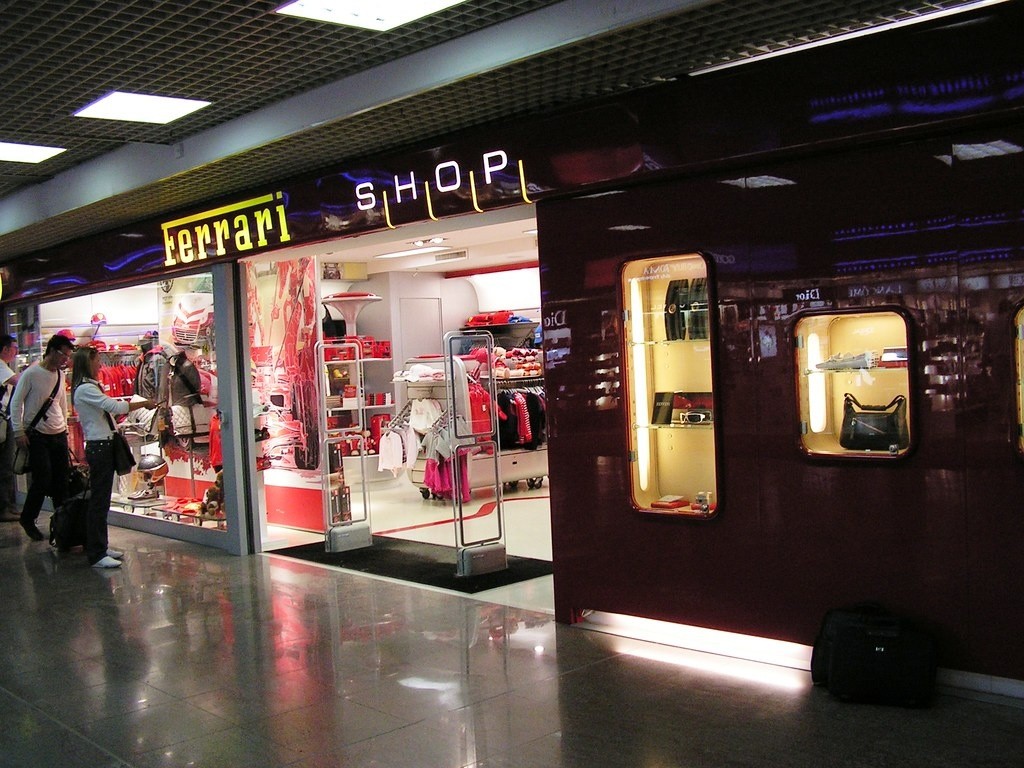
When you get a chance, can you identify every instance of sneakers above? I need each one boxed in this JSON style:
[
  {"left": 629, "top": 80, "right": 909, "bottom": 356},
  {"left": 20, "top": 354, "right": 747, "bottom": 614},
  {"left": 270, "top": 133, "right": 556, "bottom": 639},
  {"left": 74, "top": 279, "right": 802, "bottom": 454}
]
[
  {"left": 105, "top": 548, "right": 124, "bottom": 558},
  {"left": 91, "top": 555, "right": 122, "bottom": 568}
]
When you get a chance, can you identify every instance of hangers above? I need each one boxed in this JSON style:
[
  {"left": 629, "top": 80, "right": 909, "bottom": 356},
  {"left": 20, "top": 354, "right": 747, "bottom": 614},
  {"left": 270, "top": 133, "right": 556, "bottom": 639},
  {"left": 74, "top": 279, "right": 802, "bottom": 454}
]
[{"left": 98, "top": 353, "right": 139, "bottom": 366}]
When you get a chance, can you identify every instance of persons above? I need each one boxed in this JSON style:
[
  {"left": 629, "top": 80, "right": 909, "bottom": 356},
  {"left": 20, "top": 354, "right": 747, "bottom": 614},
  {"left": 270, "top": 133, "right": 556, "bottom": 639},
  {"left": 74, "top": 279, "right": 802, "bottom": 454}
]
[
  {"left": 0, "top": 335, "right": 22, "bottom": 522},
  {"left": 10, "top": 335, "right": 74, "bottom": 543},
  {"left": 71, "top": 347, "right": 157, "bottom": 568}
]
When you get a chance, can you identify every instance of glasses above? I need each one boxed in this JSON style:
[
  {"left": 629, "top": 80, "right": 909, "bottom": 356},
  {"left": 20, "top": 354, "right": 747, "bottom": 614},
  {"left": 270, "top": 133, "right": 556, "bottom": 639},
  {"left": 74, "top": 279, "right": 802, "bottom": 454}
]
[
  {"left": 55, "top": 348, "right": 71, "bottom": 361},
  {"left": 680, "top": 412, "right": 705, "bottom": 424}
]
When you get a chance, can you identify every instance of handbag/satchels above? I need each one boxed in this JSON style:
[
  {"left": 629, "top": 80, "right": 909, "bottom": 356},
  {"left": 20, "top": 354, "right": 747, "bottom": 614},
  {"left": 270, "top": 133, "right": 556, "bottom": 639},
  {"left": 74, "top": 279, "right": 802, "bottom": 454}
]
[
  {"left": 810, "top": 603, "right": 937, "bottom": 709},
  {"left": 841, "top": 393, "right": 909, "bottom": 451},
  {"left": 49, "top": 484, "right": 94, "bottom": 546},
  {"left": 0, "top": 411, "right": 8, "bottom": 443},
  {"left": 12, "top": 436, "right": 30, "bottom": 475},
  {"left": 113, "top": 432, "right": 136, "bottom": 477},
  {"left": 65, "top": 445, "right": 91, "bottom": 494},
  {"left": 127, "top": 394, "right": 160, "bottom": 433},
  {"left": 169, "top": 404, "right": 212, "bottom": 438}
]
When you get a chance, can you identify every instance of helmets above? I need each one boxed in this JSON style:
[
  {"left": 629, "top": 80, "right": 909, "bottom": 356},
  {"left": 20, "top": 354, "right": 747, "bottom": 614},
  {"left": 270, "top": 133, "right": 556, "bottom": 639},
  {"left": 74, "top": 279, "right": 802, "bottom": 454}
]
[{"left": 137, "top": 455, "right": 168, "bottom": 483}]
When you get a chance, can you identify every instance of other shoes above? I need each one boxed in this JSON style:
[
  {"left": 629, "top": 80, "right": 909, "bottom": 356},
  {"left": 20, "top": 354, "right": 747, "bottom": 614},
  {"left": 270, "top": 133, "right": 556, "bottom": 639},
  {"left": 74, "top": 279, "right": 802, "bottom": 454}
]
[
  {"left": 128, "top": 490, "right": 160, "bottom": 499},
  {"left": 815, "top": 352, "right": 872, "bottom": 370},
  {"left": 17, "top": 516, "right": 45, "bottom": 540},
  {"left": 0, "top": 508, "right": 23, "bottom": 521}
]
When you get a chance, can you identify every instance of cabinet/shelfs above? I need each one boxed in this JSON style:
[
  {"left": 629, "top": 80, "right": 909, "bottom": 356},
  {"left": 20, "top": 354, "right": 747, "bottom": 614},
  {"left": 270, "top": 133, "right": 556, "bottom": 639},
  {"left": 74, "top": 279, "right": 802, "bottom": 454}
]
[{"left": 245, "top": 255, "right": 398, "bottom": 531}]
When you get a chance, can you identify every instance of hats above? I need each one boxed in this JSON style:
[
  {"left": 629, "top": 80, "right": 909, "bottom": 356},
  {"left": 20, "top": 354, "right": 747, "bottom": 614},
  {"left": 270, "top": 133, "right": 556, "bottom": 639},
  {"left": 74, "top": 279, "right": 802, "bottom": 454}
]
[
  {"left": 58, "top": 329, "right": 76, "bottom": 341},
  {"left": 91, "top": 313, "right": 107, "bottom": 324}
]
